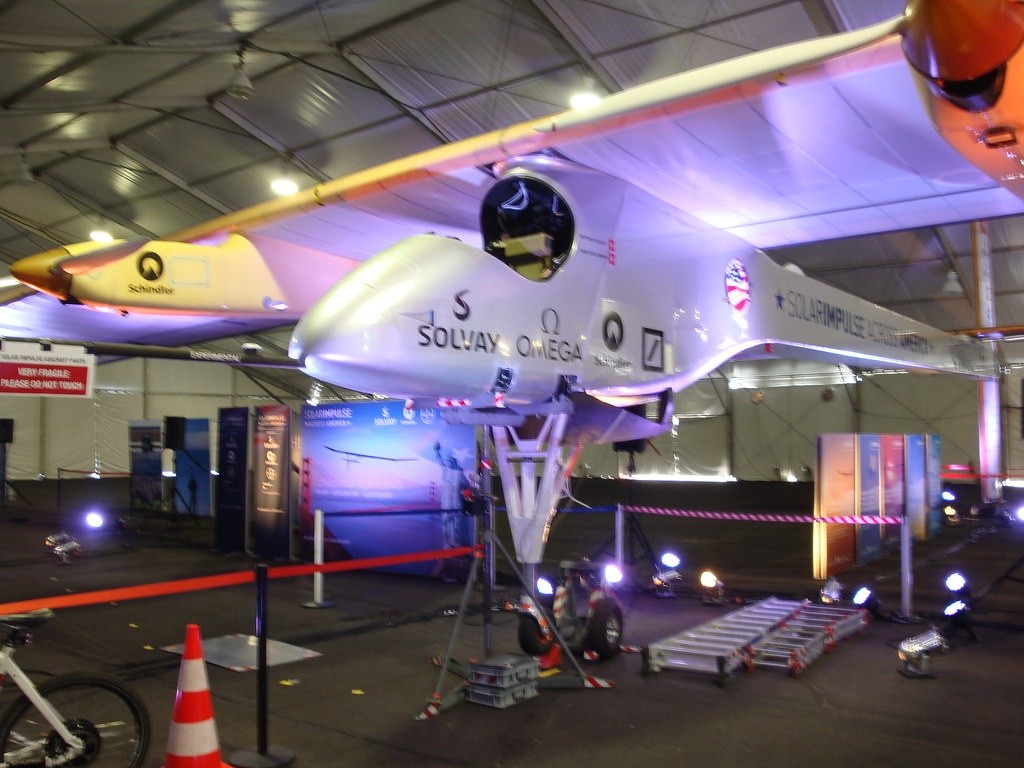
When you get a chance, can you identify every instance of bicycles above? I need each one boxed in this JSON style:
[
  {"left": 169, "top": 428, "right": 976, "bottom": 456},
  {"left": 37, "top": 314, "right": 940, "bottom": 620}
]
[{"left": 0, "top": 608, "right": 151, "bottom": 767}]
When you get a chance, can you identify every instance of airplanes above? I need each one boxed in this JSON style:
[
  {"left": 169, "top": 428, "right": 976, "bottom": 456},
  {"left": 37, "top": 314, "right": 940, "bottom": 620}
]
[{"left": 0, "top": 0, "right": 1024, "bottom": 668}]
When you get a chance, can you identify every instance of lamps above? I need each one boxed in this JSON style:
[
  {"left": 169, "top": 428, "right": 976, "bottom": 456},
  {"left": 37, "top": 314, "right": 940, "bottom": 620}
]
[
  {"left": 853, "top": 586, "right": 882, "bottom": 615},
  {"left": 699, "top": 566, "right": 723, "bottom": 604},
  {"left": 226, "top": 46, "right": 256, "bottom": 97},
  {"left": 45, "top": 531, "right": 80, "bottom": 564},
  {"left": 569, "top": 72, "right": 600, "bottom": 108},
  {"left": 820, "top": 578, "right": 844, "bottom": 605},
  {"left": 944, "top": 570, "right": 978, "bottom": 641},
  {"left": 270, "top": 161, "right": 298, "bottom": 195},
  {"left": 90, "top": 215, "right": 113, "bottom": 243},
  {"left": 652, "top": 570, "right": 682, "bottom": 597},
  {"left": 899, "top": 629, "right": 946, "bottom": 677}
]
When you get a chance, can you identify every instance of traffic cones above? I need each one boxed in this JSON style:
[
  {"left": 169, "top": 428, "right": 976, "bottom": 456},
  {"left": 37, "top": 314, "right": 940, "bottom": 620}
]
[{"left": 150, "top": 620, "right": 231, "bottom": 768}]
